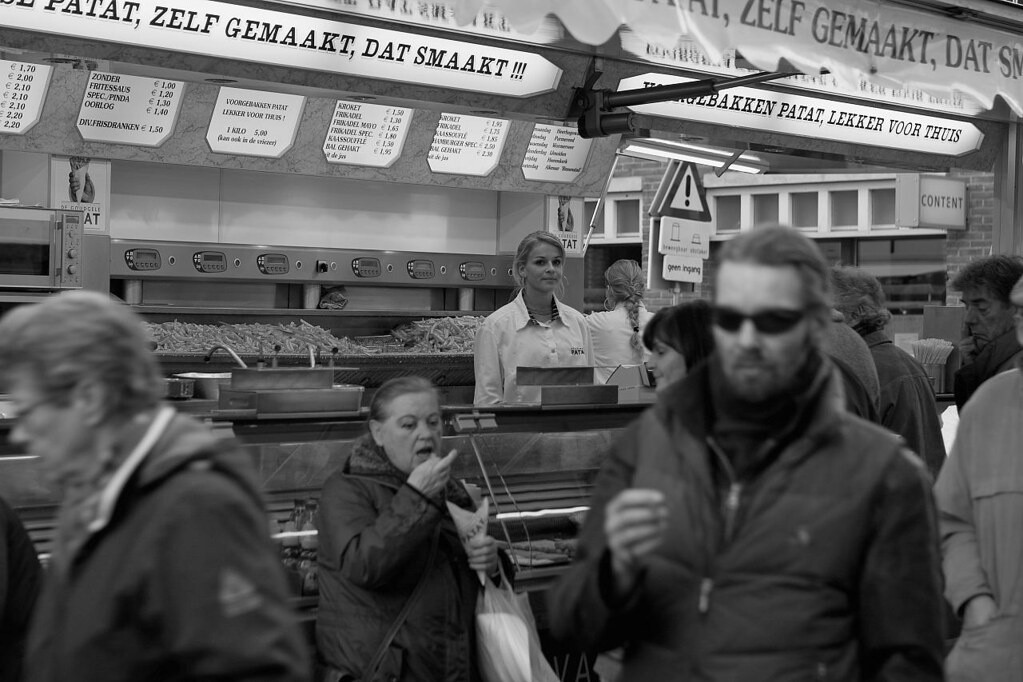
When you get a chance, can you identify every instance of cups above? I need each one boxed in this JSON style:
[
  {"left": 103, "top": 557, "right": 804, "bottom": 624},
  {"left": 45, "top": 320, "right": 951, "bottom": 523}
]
[{"left": 923, "top": 364, "right": 946, "bottom": 393}]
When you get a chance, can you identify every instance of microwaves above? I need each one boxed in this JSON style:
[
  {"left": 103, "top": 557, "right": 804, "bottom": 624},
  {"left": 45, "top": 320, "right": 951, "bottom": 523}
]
[{"left": 0, "top": 205, "right": 84, "bottom": 289}]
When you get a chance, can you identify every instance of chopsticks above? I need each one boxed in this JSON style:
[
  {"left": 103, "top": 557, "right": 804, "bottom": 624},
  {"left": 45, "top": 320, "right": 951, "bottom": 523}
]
[{"left": 912, "top": 337, "right": 955, "bottom": 364}]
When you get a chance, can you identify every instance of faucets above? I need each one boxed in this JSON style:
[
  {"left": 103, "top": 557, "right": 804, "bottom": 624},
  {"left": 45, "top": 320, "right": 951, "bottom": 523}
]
[
  {"left": 203, "top": 344, "right": 249, "bottom": 368},
  {"left": 298, "top": 344, "right": 317, "bottom": 368}
]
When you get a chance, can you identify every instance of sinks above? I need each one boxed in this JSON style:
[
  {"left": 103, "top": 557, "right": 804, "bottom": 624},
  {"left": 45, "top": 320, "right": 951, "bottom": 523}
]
[
  {"left": 334, "top": 384, "right": 365, "bottom": 411},
  {"left": 173, "top": 372, "right": 233, "bottom": 399}
]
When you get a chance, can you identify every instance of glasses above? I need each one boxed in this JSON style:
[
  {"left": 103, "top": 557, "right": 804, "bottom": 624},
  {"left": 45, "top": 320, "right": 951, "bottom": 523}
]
[{"left": 709, "top": 307, "right": 812, "bottom": 335}]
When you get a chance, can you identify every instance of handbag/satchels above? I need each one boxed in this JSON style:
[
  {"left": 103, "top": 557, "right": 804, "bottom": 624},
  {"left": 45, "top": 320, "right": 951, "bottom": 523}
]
[{"left": 473, "top": 556, "right": 559, "bottom": 682}]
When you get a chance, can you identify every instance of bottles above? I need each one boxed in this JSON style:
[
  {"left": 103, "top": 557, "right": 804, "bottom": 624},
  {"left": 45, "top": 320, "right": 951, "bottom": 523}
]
[
  {"left": 297, "top": 503, "right": 317, "bottom": 567},
  {"left": 281, "top": 499, "right": 305, "bottom": 566}
]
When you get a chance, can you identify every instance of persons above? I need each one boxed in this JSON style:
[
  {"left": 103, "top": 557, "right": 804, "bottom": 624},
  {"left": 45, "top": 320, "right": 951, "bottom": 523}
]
[
  {"left": 0, "top": 289, "right": 312, "bottom": 682},
  {"left": 316, "top": 376, "right": 515, "bottom": 682},
  {"left": 593, "top": 298, "right": 717, "bottom": 682},
  {"left": 473, "top": 231, "right": 595, "bottom": 408},
  {"left": 816, "top": 254, "right": 1023, "bottom": 682},
  {"left": 537, "top": 225, "right": 948, "bottom": 682},
  {"left": 584, "top": 259, "right": 657, "bottom": 386}
]
